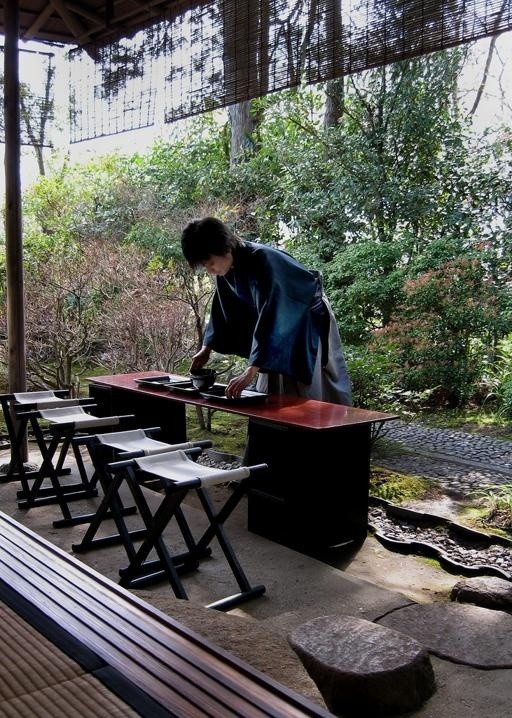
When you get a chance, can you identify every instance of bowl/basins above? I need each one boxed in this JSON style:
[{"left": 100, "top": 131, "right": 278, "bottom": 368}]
[{"left": 189, "top": 368, "right": 218, "bottom": 391}]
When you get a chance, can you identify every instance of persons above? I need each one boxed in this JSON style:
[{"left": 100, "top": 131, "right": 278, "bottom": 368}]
[{"left": 181, "top": 217, "right": 353, "bottom": 491}]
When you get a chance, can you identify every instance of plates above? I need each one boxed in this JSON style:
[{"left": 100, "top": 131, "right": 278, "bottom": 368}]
[{"left": 132, "top": 374, "right": 270, "bottom": 405}]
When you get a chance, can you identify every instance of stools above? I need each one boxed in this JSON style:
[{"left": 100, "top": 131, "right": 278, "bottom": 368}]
[
  {"left": 16, "top": 404, "right": 137, "bottom": 528},
  {"left": 1, "top": 391, "right": 95, "bottom": 497},
  {"left": 109, "top": 447, "right": 266, "bottom": 610},
  {"left": 73, "top": 427, "right": 211, "bottom": 577}
]
